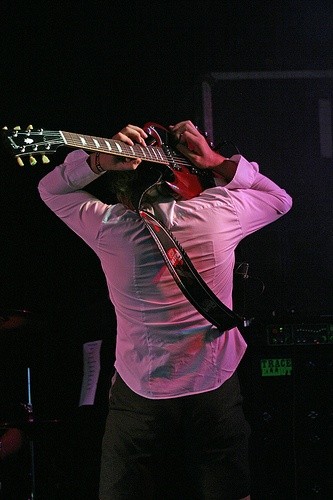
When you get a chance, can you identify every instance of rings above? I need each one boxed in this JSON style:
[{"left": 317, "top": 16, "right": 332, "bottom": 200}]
[{"left": 179, "top": 127, "right": 185, "bottom": 134}]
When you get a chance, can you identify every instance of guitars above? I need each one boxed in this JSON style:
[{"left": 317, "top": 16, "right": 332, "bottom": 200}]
[{"left": 1, "top": 119, "right": 233, "bottom": 201}]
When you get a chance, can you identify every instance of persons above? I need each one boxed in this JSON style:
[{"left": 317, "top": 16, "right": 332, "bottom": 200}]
[
  {"left": 39, "top": 121, "right": 292, "bottom": 500},
  {"left": 0, "top": 393, "right": 27, "bottom": 500}
]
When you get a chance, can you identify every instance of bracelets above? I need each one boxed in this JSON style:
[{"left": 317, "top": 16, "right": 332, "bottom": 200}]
[{"left": 95, "top": 152, "right": 106, "bottom": 175}]
